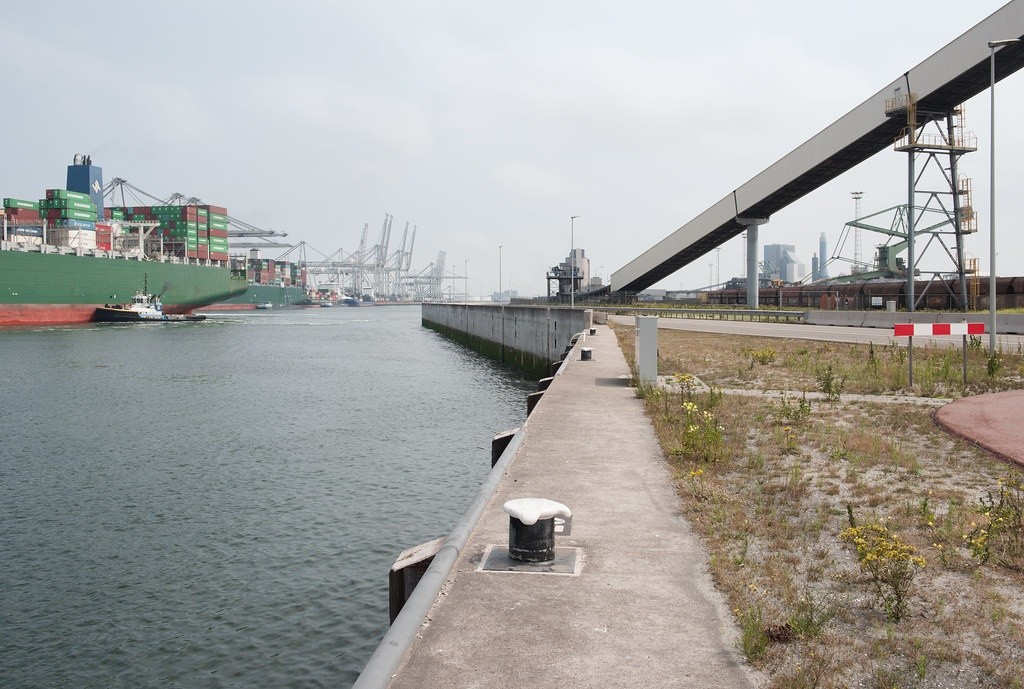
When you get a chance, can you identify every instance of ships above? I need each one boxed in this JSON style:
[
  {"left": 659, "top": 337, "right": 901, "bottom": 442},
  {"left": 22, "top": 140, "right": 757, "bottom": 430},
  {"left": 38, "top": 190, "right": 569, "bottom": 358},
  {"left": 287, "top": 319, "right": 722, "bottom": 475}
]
[{"left": 0, "top": 153, "right": 337, "bottom": 326}]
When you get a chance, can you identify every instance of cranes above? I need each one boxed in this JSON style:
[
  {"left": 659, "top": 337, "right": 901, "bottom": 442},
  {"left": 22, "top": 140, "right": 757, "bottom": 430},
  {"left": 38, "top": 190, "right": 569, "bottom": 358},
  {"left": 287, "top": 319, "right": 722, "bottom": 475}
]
[{"left": 102, "top": 177, "right": 466, "bottom": 298}]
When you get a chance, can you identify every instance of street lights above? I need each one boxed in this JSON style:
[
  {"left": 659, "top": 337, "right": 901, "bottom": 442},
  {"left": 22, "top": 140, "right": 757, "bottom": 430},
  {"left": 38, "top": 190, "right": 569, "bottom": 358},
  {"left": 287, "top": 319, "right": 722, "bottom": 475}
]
[
  {"left": 499, "top": 246, "right": 503, "bottom": 305},
  {"left": 716, "top": 248, "right": 721, "bottom": 290},
  {"left": 571, "top": 215, "right": 581, "bottom": 308},
  {"left": 742, "top": 233, "right": 748, "bottom": 278},
  {"left": 851, "top": 191, "right": 863, "bottom": 274},
  {"left": 708, "top": 263, "right": 713, "bottom": 291},
  {"left": 988, "top": 38, "right": 1021, "bottom": 358}
]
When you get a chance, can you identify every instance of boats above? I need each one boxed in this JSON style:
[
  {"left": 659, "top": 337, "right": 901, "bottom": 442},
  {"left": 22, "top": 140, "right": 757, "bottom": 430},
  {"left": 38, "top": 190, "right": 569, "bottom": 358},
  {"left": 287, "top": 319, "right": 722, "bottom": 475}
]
[
  {"left": 345, "top": 297, "right": 374, "bottom": 307},
  {"left": 96, "top": 272, "right": 206, "bottom": 322}
]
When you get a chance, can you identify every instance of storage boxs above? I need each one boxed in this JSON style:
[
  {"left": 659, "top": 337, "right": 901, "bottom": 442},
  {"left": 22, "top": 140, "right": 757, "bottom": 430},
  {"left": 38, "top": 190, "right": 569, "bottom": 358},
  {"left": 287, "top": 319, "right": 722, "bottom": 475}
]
[
  {"left": 3, "top": 189, "right": 227, "bottom": 259},
  {"left": 232, "top": 257, "right": 307, "bottom": 286},
  {"left": 305, "top": 290, "right": 339, "bottom": 302}
]
